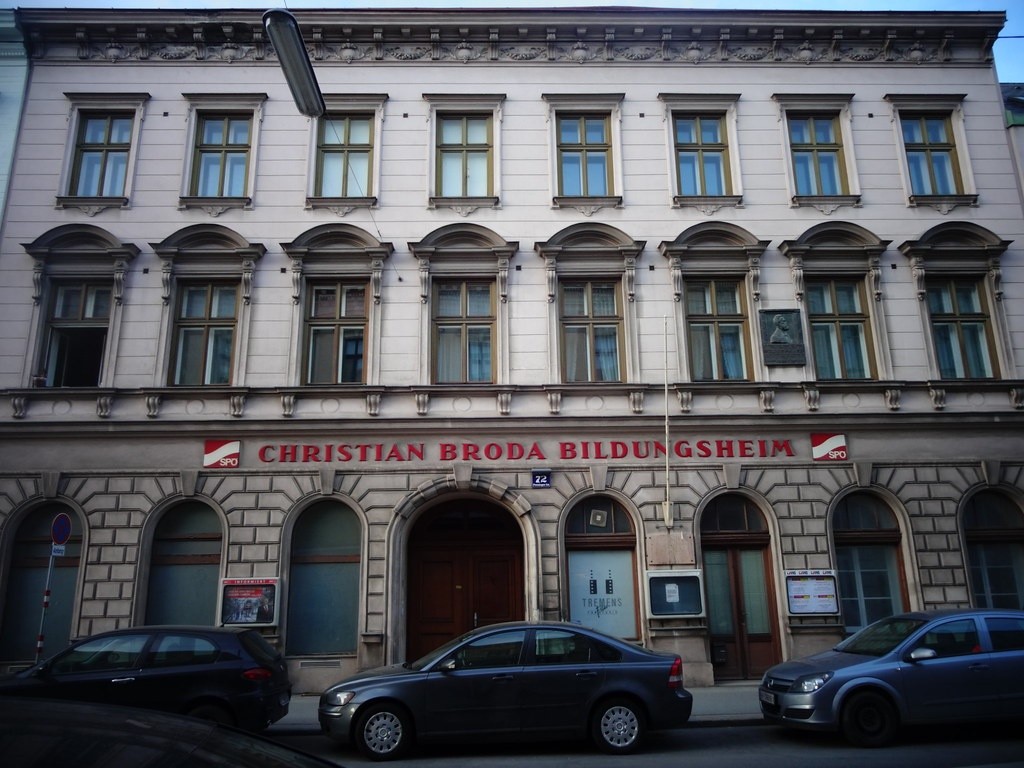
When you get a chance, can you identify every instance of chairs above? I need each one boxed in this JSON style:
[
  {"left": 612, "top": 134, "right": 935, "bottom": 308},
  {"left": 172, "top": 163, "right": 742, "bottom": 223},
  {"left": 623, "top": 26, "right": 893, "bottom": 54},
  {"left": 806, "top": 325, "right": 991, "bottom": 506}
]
[{"left": 128, "top": 615, "right": 1023, "bottom": 667}]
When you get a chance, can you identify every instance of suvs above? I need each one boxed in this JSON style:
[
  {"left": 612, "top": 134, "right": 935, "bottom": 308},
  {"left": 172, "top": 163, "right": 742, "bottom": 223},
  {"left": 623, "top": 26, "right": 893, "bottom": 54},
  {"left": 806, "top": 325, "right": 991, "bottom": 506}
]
[{"left": 1, "top": 625, "right": 293, "bottom": 734}]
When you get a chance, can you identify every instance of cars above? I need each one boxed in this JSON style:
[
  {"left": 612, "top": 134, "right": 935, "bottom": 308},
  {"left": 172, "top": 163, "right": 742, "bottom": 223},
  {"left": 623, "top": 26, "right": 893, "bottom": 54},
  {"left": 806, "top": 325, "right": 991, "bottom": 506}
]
[
  {"left": 317, "top": 620, "right": 693, "bottom": 763},
  {"left": 0, "top": 698, "right": 345, "bottom": 768},
  {"left": 758, "top": 608, "right": 1024, "bottom": 749}
]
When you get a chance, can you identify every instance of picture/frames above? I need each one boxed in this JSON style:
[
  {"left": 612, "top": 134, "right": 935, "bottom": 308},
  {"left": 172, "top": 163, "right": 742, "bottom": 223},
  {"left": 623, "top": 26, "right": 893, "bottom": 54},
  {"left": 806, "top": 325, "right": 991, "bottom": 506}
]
[{"left": 216, "top": 578, "right": 280, "bottom": 628}]
